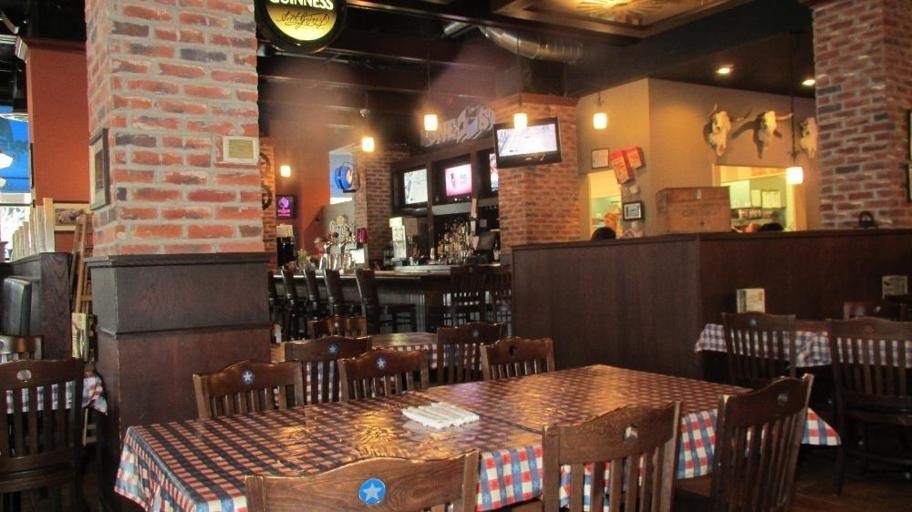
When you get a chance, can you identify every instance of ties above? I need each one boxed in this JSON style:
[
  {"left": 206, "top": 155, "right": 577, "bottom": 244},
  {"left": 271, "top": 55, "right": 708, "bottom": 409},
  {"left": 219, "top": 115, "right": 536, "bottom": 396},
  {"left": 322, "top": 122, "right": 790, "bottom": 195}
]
[
  {"left": 495, "top": 117, "right": 562, "bottom": 169},
  {"left": 480, "top": 152, "right": 498, "bottom": 198},
  {"left": 436, "top": 159, "right": 472, "bottom": 204},
  {"left": 400, "top": 167, "right": 427, "bottom": 206}
]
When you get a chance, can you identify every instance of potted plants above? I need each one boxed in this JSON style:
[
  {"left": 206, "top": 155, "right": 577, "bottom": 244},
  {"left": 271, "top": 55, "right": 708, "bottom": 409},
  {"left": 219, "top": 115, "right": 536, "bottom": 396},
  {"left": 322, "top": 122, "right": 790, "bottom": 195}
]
[
  {"left": 623, "top": 201, "right": 643, "bottom": 220},
  {"left": 591, "top": 148, "right": 609, "bottom": 169},
  {"left": 87, "top": 128, "right": 110, "bottom": 210},
  {"left": 223, "top": 136, "right": 260, "bottom": 164}
]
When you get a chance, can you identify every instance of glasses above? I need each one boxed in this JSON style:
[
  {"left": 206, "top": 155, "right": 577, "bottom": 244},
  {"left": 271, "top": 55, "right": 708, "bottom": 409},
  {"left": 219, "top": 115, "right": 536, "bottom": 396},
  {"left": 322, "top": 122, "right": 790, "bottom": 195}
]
[{"left": 786, "top": 38, "right": 803, "bottom": 185}]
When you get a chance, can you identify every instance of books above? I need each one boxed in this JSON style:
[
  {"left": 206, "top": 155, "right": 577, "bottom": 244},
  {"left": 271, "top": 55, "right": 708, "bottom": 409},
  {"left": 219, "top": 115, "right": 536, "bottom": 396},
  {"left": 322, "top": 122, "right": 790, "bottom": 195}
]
[{"left": 655, "top": 186, "right": 731, "bottom": 237}]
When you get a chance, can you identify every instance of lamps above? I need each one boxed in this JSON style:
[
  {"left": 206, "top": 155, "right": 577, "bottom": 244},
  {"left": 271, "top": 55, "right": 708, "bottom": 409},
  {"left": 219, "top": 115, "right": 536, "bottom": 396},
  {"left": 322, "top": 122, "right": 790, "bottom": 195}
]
[
  {"left": 786, "top": 38, "right": 803, "bottom": 185},
  {"left": 281, "top": 90, "right": 293, "bottom": 176},
  {"left": 513, "top": 28, "right": 527, "bottom": 132},
  {"left": 194, "top": 359, "right": 304, "bottom": 418},
  {"left": 361, "top": 56, "right": 375, "bottom": 154},
  {"left": 268, "top": 264, "right": 555, "bottom": 401},
  {"left": 243, "top": 447, "right": 483, "bottom": 511},
  {"left": 1, "top": 359, "right": 85, "bottom": 512},
  {"left": 542, "top": 399, "right": 682, "bottom": 511},
  {"left": 709, "top": 373, "right": 814, "bottom": 512},
  {"left": 423, "top": 28, "right": 440, "bottom": 132},
  {"left": 592, "top": 27, "right": 607, "bottom": 131}
]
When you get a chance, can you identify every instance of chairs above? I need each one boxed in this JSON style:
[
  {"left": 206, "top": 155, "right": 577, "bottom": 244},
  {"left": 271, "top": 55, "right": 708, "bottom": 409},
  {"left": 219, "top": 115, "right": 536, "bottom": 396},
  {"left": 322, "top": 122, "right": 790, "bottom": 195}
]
[
  {"left": 1, "top": 359, "right": 85, "bottom": 512},
  {"left": 542, "top": 399, "right": 682, "bottom": 511},
  {"left": 268, "top": 264, "right": 555, "bottom": 401},
  {"left": 243, "top": 447, "right": 483, "bottom": 511},
  {"left": 694, "top": 301, "right": 912, "bottom": 494},
  {"left": 709, "top": 373, "right": 814, "bottom": 512},
  {"left": 194, "top": 359, "right": 304, "bottom": 418}
]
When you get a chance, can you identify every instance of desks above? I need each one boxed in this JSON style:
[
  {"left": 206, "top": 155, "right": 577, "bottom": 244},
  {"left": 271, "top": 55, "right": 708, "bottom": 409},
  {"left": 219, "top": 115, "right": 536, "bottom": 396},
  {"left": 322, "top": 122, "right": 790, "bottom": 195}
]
[{"left": 3, "top": 369, "right": 108, "bottom": 459}]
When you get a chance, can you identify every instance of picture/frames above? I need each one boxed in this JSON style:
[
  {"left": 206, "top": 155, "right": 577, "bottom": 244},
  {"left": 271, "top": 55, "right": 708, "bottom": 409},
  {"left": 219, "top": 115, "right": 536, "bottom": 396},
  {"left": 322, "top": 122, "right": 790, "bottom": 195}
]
[
  {"left": 3, "top": 369, "right": 108, "bottom": 459},
  {"left": 87, "top": 128, "right": 110, "bottom": 210},
  {"left": 223, "top": 136, "right": 260, "bottom": 164},
  {"left": 623, "top": 201, "right": 643, "bottom": 220},
  {"left": 591, "top": 148, "right": 609, "bottom": 169}
]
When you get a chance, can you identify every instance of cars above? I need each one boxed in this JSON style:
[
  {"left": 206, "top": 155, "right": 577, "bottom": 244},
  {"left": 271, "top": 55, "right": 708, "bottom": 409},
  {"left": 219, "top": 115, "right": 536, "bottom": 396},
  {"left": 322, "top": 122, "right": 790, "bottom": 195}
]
[{"left": 319, "top": 253, "right": 356, "bottom": 275}]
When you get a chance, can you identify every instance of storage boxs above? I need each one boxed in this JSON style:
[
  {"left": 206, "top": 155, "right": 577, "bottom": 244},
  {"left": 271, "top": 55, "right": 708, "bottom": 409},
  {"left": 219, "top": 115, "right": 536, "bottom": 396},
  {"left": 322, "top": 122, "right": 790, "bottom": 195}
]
[{"left": 655, "top": 186, "right": 731, "bottom": 237}]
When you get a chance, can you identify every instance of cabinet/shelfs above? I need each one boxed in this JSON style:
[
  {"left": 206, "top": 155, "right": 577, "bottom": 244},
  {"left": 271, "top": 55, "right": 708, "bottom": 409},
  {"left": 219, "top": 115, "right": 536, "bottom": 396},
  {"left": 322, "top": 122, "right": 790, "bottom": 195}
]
[{"left": 429, "top": 215, "right": 470, "bottom": 266}]
